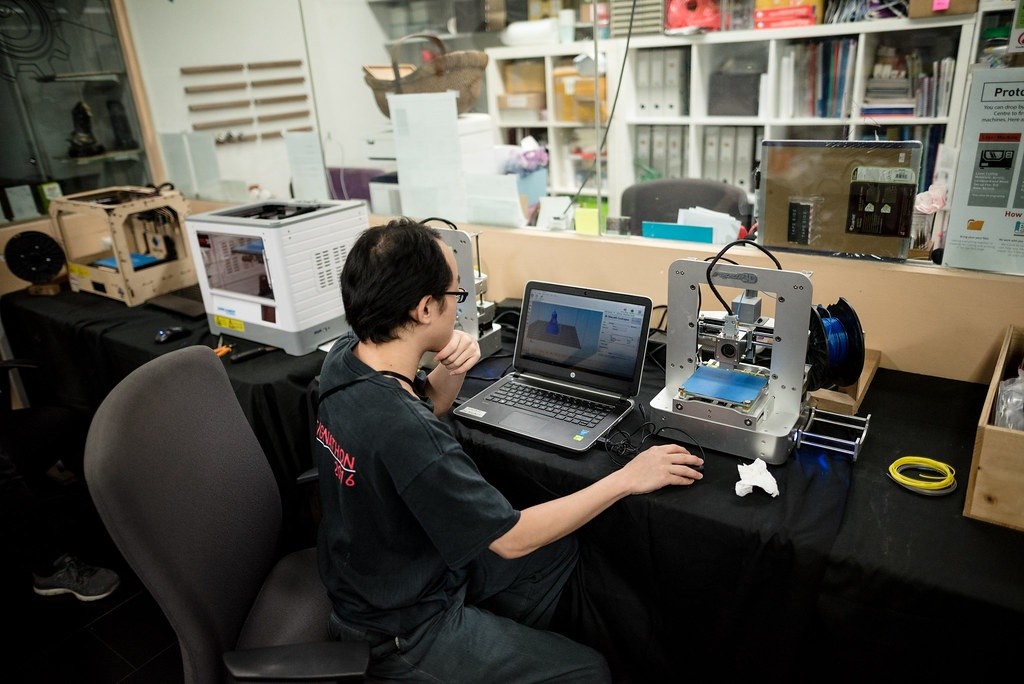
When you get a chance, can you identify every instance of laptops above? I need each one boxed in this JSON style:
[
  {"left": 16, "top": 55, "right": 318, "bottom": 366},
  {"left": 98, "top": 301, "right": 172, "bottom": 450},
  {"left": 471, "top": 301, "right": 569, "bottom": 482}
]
[
  {"left": 452, "top": 281, "right": 653, "bottom": 451},
  {"left": 146, "top": 283, "right": 207, "bottom": 322}
]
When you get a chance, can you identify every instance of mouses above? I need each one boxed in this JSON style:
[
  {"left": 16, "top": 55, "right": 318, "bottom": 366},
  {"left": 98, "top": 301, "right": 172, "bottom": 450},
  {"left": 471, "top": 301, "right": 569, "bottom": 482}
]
[
  {"left": 154, "top": 326, "right": 193, "bottom": 344},
  {"left": 677, "top": 463, "right": 704, "bottom": 480}
]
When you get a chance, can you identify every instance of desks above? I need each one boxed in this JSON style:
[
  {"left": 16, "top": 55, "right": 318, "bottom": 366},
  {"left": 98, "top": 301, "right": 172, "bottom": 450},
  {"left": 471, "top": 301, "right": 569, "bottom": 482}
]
[
  {"left": 0, "top": 275, "right": 329, "bottom": 560},
  {"left": 307, "top": 299, "right": 1024, "bottom": 684}
]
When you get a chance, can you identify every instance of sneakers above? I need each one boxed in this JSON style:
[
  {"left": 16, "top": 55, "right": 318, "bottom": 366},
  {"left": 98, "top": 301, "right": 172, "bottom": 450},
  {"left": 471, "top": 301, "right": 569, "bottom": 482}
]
[{"left": 31, "top": 559, "right": 121, "bottom": 600}]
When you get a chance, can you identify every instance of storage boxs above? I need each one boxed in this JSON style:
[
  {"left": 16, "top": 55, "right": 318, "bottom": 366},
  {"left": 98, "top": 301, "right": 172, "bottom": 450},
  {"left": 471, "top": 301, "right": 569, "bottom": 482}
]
[
  {"left": 575, "top": 77, "right": 605, "bottom": 102},
  {"left": 497, "top": 93, "right": 545, "bottom": 109},
  {"left": 554, "top": 67, "right": 579, "bottom": 122},
  {"left": 505, "top": 61, "right": 546, "bottom": 93},
  {"left": 578, "top": 100, "right": 607, "bottom": 121},
  {"left": 501, "top": 109, "right": 541, "bottom": 122}
]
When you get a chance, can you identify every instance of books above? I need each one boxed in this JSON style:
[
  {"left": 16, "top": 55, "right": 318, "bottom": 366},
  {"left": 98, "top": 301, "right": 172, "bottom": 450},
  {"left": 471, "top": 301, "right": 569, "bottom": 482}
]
[{"left": 780, "top": 37, "right": 956, "bottom": 265}]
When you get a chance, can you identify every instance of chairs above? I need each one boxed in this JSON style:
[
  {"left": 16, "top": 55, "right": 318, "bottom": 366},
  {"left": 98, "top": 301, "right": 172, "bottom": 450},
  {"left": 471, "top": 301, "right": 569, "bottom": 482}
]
[
  {"left": 83, "top": 344, "right": 373, "bottom": 684},
  {"left": 620, "top": 176, "right": 754, "bottom": 246}
]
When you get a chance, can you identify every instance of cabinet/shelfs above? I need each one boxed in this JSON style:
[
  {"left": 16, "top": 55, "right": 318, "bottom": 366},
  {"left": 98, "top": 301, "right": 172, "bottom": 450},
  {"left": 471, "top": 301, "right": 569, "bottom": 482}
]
[{"left": 483, "top": 15, "right": 976, "bottom": 258}]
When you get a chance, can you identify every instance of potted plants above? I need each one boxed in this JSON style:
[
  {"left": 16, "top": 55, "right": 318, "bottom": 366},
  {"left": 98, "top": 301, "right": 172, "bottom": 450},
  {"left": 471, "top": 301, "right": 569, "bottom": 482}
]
[{"left": 574, "top": 197, "right": 609, "bottom": 235}]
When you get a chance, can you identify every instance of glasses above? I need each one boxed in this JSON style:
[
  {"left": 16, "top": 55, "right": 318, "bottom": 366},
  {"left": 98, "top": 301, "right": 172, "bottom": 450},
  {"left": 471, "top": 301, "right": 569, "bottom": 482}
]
[{"left": 441, "top": 287, "right": 468, "bottom": 303}]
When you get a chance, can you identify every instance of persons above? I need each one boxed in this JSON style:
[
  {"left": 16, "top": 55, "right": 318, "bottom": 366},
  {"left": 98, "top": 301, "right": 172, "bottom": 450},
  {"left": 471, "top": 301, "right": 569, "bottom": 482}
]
[
  {"left": 0, "top": 447, "right": 120, "bottom": 601},
  {"left": 314, "top": 218, "right": 704, "bottom": 684}
]
